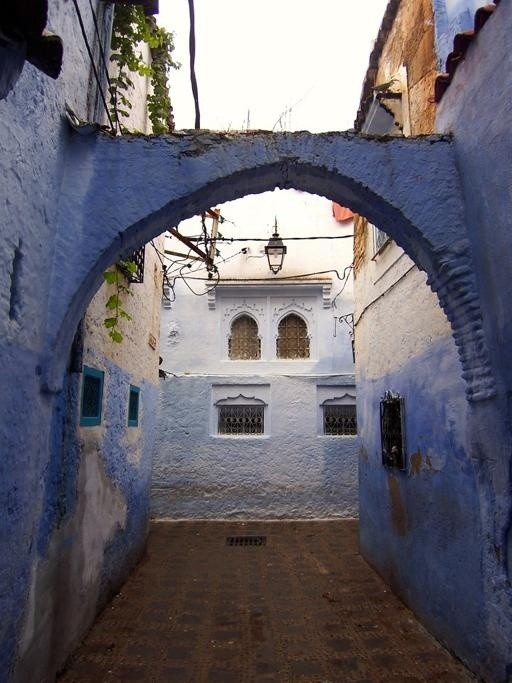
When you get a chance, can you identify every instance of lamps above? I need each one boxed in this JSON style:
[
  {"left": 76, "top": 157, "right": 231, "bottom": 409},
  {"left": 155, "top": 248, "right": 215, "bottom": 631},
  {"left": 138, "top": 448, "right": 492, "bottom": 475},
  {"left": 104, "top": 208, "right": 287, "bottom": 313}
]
[{"left": 264, "top": 217, "right": 287, "bottom": 275}]
